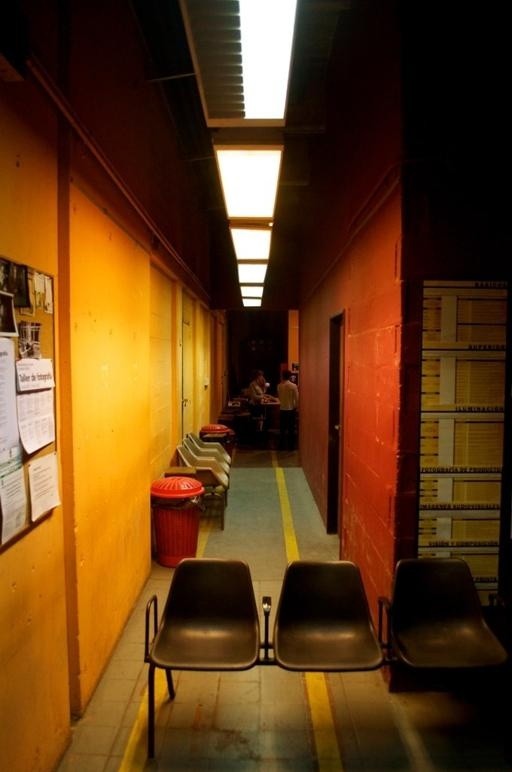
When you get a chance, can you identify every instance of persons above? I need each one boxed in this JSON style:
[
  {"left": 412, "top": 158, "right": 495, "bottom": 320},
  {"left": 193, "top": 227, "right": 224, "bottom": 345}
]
[
  {"left": 276, "top": 369, "right": 300, "bottom": 452},
  {"left": 247, "top": 369, "right": 276, "bottom": 438}
]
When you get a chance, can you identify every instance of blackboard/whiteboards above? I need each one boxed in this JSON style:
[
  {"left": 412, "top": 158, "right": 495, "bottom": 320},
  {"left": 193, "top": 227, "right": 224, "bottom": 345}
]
[{"left": 0, "top": 256, "right": 56, "bottom": 554}]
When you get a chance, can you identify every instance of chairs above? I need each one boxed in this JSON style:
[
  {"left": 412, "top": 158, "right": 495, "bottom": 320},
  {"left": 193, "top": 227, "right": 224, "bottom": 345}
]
[
  {"left": 138, "top": 559, "right": 511, "bottom": 771},
  {"left": 175, "top": 431, "right": 232, "bottom": 531}
]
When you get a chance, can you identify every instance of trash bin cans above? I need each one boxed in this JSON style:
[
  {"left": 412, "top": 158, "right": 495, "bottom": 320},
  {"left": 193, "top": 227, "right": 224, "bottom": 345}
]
[
  {"left": 200, "top": 424, "right": 235, "bottom": 457},
  {"left": 151, "top": 476, "right": 205, "bottom": 567}
]
